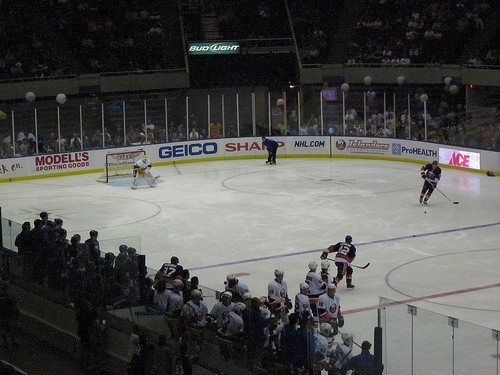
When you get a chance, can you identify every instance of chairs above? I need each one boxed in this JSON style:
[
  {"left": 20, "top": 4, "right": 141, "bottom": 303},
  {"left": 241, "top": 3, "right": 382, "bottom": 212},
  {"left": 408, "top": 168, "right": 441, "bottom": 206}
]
[{"left": 160, "top": 311, "right": 339, "bottom": 375}]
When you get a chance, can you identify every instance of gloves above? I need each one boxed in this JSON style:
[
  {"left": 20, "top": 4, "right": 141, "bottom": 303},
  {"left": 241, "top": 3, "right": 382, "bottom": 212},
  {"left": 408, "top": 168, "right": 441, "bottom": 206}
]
[
  {"left": 287, "top": 299, "right": 292, "bottom": 309},
  {"left": 308, "top": 315, "right": 315, "bottom": 326},
  {"left": 321, "top": 252, "right": 328, "bottom": 260},
  {"left": 432, "top": 182, "right": 436, "bottom": 188},
  {"left": 326, "top": 316, "right": 345, "bottom": 328},
  {"left": 325, "top": 363, "right": 332, "bottom": 371},
  {"left": 421, "top": 172, "right": 426, "bottom": 179}
]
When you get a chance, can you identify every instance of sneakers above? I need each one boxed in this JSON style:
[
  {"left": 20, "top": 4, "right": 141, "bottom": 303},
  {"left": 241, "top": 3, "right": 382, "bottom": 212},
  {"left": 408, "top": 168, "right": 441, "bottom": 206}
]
[
  {"left": 420, "top": 196, "right": 428, "bottom": 205},
  {"left": 346, "top": 279, "right": 355, "bottom": 290}
]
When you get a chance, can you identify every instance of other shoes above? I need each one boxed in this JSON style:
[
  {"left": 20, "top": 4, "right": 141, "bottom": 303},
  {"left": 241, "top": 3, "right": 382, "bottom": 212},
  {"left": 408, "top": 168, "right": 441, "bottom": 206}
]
[
  {"left": 188, "top": 354, "right": 200, "bottom": 362},
  {"left": 266, "top": 161, "right": 276, "bottom": 165}
]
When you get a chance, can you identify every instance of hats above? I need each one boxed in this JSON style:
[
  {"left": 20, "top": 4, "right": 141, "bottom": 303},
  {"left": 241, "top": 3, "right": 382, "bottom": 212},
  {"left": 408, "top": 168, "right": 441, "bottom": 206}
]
[{"left": 251, "top": 297, "right": 261, "bottom": 307}]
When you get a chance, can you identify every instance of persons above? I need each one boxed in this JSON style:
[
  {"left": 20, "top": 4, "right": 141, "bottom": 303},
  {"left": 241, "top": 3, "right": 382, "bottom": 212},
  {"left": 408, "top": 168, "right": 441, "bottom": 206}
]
[
  {"left": 261, "top": 136, "right": 279, "bottom": 166},
  {"left": 419, "top": 161, "right": 441, "bottom": 207},
  {"left": 0, "top": 210, "right": 384, "bottom": 375},
  {"left": 0, "top": 0, "right": 500, "bottom": 159},
  {"left": 132, "top": 155, "right": 157, "bottom": 189}
]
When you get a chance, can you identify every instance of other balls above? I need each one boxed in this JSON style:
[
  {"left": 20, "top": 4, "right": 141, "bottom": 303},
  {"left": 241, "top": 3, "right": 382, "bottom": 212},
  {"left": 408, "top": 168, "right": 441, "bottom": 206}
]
[
  {"left": 424, "top": 211, "right": 426, "bottom": 213},
  {"left": 424, "top": 176, "right": 459, "bottom": 204}
]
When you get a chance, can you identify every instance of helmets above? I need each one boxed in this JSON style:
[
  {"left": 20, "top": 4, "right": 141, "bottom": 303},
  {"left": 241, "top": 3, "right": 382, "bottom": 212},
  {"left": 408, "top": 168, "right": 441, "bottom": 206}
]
[
  {"left": 235, "top": 302, "right": 247, "bottom": 311},
  {"left": 341, "top": 333, "right": 352, "bottom": 341},
  {"left": 172, "top": 279, "right": 184, "bottom": 292},
  {"left": 191, "top": 289, "right": 201, "bottom": 298},
  {"left": 227, "top": 274, "right": 236, "bottom": 282},
  {"left": 299, "top": 282, "right": 310, "bottom": 291},
  {"left": 221, "top": 291, "right": 232, "bottom": 300},
  {"left": 345, "top": 236, "right": 352, "bottom": 242},
  {"left": 308, "top": 260, "right": 330, "bottom": 270},
  {"left": 243, "top": 293, "right": 253, "bottom": 299},
  {"left": 320, "top": 323, "right": 333, "bottom": 337},
  {"left": 328, "top": 283, "right": 336, "bottom": 289},
  {"left": 433, "top": 161, "right": 437, "bottom": 165},
  {"left": 260, "top": 296, "right": 267, "bottom": 304},
  {"left": 274, "top": 268, "right": 284, "bottom": 277}
]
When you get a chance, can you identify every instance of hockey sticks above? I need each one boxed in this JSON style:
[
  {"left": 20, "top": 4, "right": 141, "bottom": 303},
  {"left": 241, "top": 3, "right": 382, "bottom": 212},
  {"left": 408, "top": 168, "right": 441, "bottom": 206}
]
[
  {"left": 142, "top": 173, "right": 161, "bottom": 185},
  {"left": 326, "top": 257, "right": 370, "bottom": 269}
]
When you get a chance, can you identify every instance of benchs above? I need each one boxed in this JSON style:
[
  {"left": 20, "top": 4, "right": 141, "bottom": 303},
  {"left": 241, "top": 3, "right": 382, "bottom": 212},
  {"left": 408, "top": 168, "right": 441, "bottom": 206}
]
[{"left": 0, "top": 103, "right": 167, "bottom": 145}]
